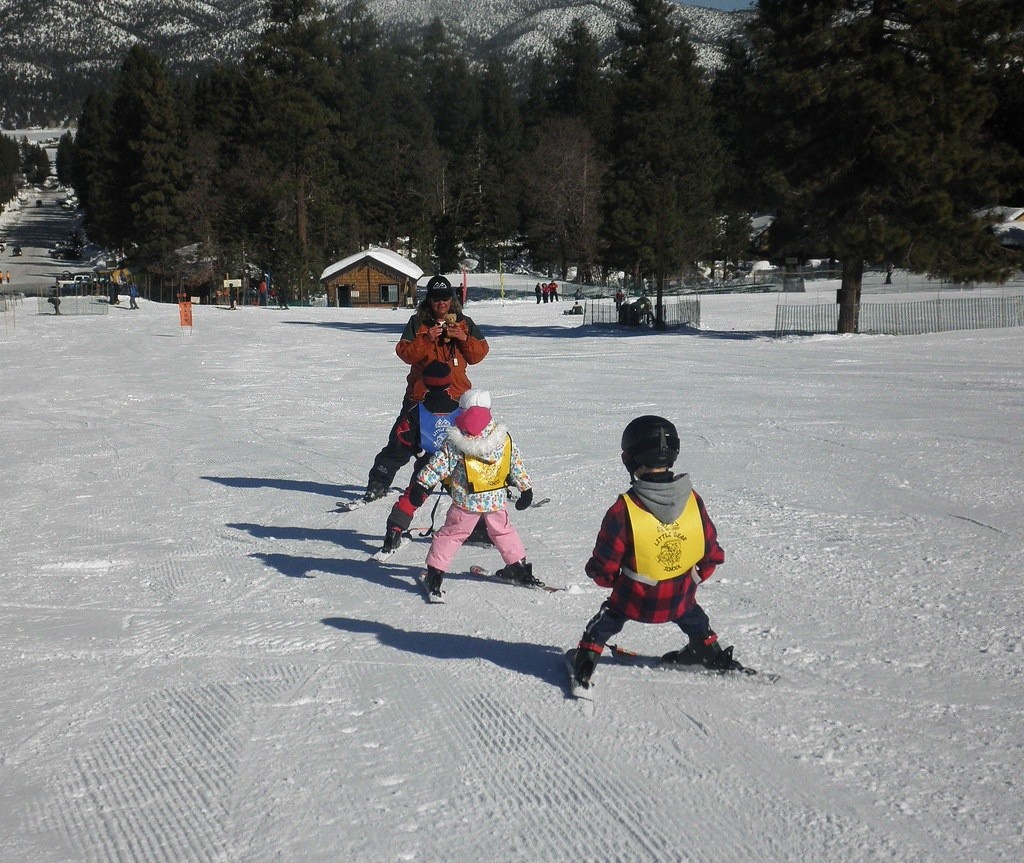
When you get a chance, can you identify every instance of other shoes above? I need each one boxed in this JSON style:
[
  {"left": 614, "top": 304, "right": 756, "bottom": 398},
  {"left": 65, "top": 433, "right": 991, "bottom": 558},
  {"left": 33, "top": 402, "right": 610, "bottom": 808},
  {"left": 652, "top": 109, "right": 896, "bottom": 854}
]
[
  {"left": 383, "top": 527, "right": 402, "bottom": 553},
  {"left": 496, "top": 559, "right": 532, "bottom": 583},
  {"left": 364, "top": 481, "right": 385, "bottom": 502},
  {"left": 663, "top": 641, "right": 725, "bottom": 668},
  {"left": 571, "top": 647, "right": 597, "bottom": 684},
  {"left": 425, "top": 566, "right": 444, "bottom": 595}
]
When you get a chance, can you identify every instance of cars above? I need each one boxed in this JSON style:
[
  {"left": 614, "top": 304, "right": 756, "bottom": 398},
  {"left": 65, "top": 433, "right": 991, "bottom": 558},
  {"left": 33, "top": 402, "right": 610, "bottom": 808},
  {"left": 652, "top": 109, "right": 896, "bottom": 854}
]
[
  {"left": 0, "top": 238, "right": 8, "bottom": 253},
  {"left": 49, "top": 241, "right": 83, "bottom": 260},
  {"left": 972, "top": 206, "right": 1024, "bottom": 245},
  {"left": 37, "top": 136, "right": 79, "bottom": 210},
  {"left": 13, "top": 248, "right": 23, "bottom": 256}
]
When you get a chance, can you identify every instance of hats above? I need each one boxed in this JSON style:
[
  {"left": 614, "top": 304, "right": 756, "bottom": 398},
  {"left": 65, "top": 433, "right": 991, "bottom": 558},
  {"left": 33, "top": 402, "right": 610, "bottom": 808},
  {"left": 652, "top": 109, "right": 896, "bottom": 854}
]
[
  {"left": 422, "top": 359, "right": 451, "bottom": 389},
  {"left": 459, "top": 387, "right": 491, "bottom": 412},
  {"left": 426, "top": 275, "right": 455, "bottom": 292}
]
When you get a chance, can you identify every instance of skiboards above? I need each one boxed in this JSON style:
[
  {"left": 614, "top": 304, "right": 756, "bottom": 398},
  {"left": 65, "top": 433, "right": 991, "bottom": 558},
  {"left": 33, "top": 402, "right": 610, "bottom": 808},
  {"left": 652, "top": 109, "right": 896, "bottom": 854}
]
[
  {"left": 567, "top": 648, "right": 779, "bottom": 701},
  {"left": 336, "top": 486, "right": 403, "bottom": 510},
  {"left": 362, "top": 530, "right": 412, "bottom": 568},
  {"left": 419, "top": 566, "right": 565, "bottom": 603}
]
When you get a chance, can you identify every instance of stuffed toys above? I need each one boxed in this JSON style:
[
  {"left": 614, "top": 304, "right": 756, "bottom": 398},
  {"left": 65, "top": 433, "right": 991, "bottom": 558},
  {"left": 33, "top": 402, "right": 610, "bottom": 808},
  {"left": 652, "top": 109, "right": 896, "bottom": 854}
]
[{"left": 441, "top": 314, "right": 461, "bottom": 345}]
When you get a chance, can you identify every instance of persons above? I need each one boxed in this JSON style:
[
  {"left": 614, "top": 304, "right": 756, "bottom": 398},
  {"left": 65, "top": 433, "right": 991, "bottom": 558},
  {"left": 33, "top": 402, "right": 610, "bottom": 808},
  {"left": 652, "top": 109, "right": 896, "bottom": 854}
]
[
  {"left": 364, "top": 276, "right": 490, "bottom": 502},
  {"left": 615, "top": 289, "right": 624, "bottom": 310},
  {"left": 535, "top": 280, "right": 559, "bottom": 304},
  {"left": 567, "top": 414, "right": 734, "bottom": 684},
  {"left": 636, "top": 291, "right": 654, "bottom": 323},
  {"left": 572, "top": 301, "right": 583, "bottom": 314},
  {"left": 382, "top": 360, "right": 494, "bottom": 548},
  {"left": 410, "top": 389, "right": 533, "bottom": 596},
  {"left": 0, "top": 271, "right": 140, "bottom": 311},
  {"left": 884, "top": 262, "right": 894, "bottom": 283},
  {"left": 216, "top": 278, "right": 289, "bottom": 311},
  {"left": 456, "top": 283, "right": 464, "bottom": 306}
]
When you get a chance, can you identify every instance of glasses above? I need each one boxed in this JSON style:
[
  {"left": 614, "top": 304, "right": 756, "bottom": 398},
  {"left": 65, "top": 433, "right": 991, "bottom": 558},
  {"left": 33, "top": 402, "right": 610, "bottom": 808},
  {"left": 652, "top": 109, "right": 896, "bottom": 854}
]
[{"left": 430, "top": 294, "right": 453, "bottom": 302}]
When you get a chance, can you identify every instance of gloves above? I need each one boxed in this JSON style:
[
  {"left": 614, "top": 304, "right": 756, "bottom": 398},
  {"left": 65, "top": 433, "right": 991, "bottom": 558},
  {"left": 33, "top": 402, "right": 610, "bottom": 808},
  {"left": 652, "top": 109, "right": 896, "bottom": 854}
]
[
  {"left": 409, "top": 482, "right": 426, "bottom": 506},
  {"left": 515, "top": 488, "right": 532, "bottom": 510}
]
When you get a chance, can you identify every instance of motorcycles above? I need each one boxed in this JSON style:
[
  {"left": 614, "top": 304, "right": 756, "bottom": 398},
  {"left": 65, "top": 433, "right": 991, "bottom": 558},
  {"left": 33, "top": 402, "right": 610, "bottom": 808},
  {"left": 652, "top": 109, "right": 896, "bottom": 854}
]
[{"left": 620, "top": 293, "right": 656, "bottom": 326}]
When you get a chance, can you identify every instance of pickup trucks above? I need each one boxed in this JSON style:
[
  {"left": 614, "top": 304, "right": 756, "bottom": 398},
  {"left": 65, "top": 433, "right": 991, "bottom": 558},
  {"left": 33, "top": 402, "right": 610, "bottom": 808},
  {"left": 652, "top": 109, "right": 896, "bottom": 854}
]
[{"left": 57, "top": 275, "right": 107, "bottom": 289}]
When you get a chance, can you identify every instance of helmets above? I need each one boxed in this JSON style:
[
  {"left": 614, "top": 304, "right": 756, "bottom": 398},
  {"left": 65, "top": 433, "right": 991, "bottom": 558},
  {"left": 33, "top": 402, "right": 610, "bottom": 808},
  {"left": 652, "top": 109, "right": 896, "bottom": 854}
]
[{"left": 621, "top": 414, "right": 680, "bottom": 473}]
